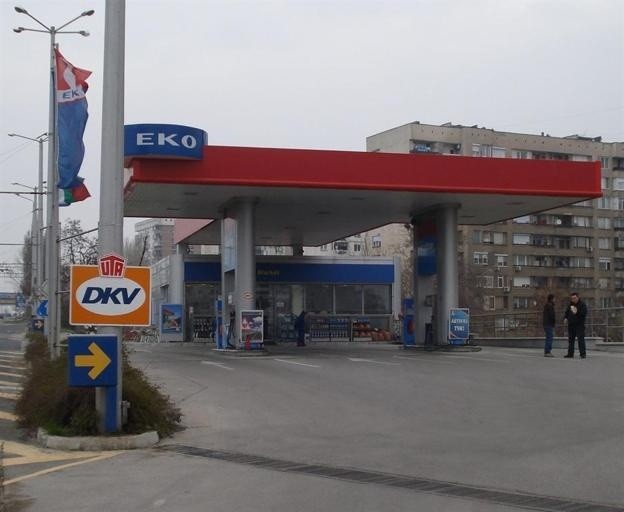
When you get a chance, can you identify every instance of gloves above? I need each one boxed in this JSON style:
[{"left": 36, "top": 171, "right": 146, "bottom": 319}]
[
  {"left": 564, "top": 319, "right": 568, "bottom": 324},
  {"left": 571, "top": 306, "right": 578, "bottom": 314}
]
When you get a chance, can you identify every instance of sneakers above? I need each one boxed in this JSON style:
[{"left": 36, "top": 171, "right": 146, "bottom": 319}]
[{"left": 544, "top": 351, "right": 586, "bottom": 358}]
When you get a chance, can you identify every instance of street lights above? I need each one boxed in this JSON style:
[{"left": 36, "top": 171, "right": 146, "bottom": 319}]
[{"left": 7, "top": 3, "right": 95, "bottom": 353}]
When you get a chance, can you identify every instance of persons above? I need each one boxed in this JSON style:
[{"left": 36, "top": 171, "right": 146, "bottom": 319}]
[
  {"left": 563, "top": 292, "right": 587, "bottom": 359},
  {"left": 294, "top": 311, "right": 307, "bottom": 346},
  {"left": 542, "top": 294, "right": 556, "bottom": 357}
]
